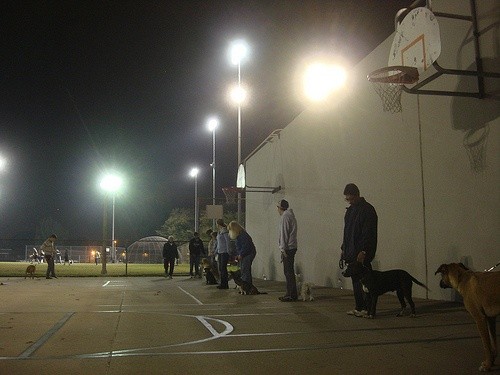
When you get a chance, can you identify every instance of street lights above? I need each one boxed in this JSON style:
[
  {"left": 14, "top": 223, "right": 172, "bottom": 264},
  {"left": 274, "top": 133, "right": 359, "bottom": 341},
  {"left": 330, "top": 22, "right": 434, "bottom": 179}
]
[
  {"left": 206, "top": 117, "right": 222, "bottom": 234},
  {"left": 190, "top": 167, "right": 200, "bottom": 232},
  {"left": 102, "top": 173, "right": 122, "bottom": 263},
  {"left": 225, "top": 40, "right": 251, "bottom": 226}
]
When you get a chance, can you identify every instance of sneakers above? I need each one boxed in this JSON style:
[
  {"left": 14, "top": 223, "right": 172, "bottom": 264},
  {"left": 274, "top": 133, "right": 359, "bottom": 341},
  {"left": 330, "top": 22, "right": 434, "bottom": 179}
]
[
  {"left": 348, "top": 309, "right": 359, "bottom": 314},
  {"left": 355, "top": 310, "right": 367, "bottom": 317}
]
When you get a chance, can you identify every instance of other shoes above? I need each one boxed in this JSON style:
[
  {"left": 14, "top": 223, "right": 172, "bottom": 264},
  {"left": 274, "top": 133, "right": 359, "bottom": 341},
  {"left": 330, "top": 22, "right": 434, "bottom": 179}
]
[
  {"left": 165, "top": 272, "right": 167, "bottom": 277},
  {"left": 195, "top": 275, "right": 199, "bottom": 278},
  {"left": 190, "top": 275, "right": 193, "bottom": 279},
  {"left": 216, "top": 286, "right": 223, "bottom": 289},
  {"left": 53, "top": 274, "right": 57, "bottom": 278},
  {"left": 47, "top": 277, "right": 53, "bottom": 279},
  {"left": 169, "top": 274, "right": 172, "bottom": 279},
  {"left": 278, "top": 296, "right": 298, "bottom": 302}
]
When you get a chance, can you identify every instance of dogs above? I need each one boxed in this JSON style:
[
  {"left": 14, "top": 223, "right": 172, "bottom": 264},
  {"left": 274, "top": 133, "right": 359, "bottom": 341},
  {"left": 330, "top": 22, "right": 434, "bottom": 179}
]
[
  {"left": 233, "top": 277, "right": 268, "bottom": 295},
  {"left": 228, "top": 270, "right": 240, "bottom": 280},
  {"left": 25, "top": 265, "right": 36, "bottom": 279},
  {"left": 342, "top": 263, "right": 431, "bottom": 319},
  {"left": 204, "top": 268, "right": 219, "bottom": 285},
  {"left": 295, "top": 273, "right": 315, "bottom": 302},
  {"left": 434, "top": 263, "right": 500, "bottom": 373},
  {"left": 200, "top": 258, "right": 219, "bottom": 281}
]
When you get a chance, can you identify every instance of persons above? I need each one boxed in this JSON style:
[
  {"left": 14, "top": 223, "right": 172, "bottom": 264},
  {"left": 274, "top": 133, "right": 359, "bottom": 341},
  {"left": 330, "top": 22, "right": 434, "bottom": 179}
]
[
  {"left": 54, "top": 249, "right": 62, "bottom": 265},
  {"left": 64, "top": 248, "right": 71, "bottom": 266},
  {"left": 228, "top": 220, "right": 257, "bottom": 295},
  {"left": 30, "top": 247, "right": 47, "bottom": 264},
  {"left": 213, "top": 219, "right": 235, "bottom": 289},
  {"left": 95, "top": 250, "right": 100, "bottom": 265},
  {"left": 189, "top": 232, "right": 205, "bottom": 277},
  {"left": 162, "top": 235, "right": 179, "bottom": 279},
  {"left": 41, "top": 233, "right": 59, "bottom": 280},
  {"left": 341, "top": 183, "right": 379, "bottom": 318},
  {"left": 206, "top": 229, "right": 220, "bottom": 281},
  {"left": 276, "top": 199, "right": 299, "bottom": 302}
]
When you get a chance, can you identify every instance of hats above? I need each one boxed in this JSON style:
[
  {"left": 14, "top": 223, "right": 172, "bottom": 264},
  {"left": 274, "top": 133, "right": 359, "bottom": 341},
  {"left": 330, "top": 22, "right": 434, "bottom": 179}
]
[
  {"left": 277, "top": 199, "right": 289, "bottom": 210},
  {"left": 344, "top": 183, "right": 360, "bottom": 195}
]
